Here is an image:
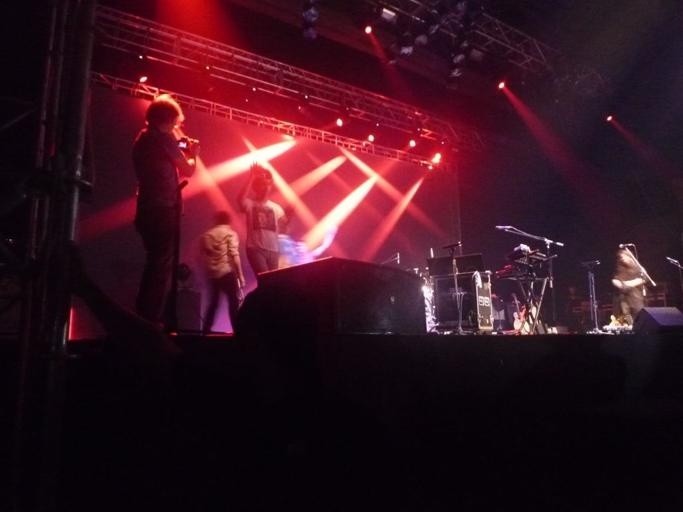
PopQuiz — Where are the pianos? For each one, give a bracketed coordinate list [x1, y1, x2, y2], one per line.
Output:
[498, 248, 546, 279]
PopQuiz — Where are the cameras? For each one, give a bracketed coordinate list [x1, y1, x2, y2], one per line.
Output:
[177, 137, 190, 150]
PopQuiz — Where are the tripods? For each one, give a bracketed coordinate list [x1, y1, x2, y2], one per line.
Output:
[587, 273, 613, 334]
[441, 249, 474, 335]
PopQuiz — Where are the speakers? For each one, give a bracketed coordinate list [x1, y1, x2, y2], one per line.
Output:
[257, 257, 426, 334]
[631, 306, 683, 334]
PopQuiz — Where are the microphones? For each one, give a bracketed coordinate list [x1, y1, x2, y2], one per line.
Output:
[665, 256, 680, 264]
[619, 243, 633, 248]
[496, 226, 512, 230]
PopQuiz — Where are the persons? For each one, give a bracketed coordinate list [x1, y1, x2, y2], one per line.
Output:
[611, 248, 650, 332]
[129, 95, 200, 335]
[202, 210, 246, 333]
[236, 161, 287, 277]
